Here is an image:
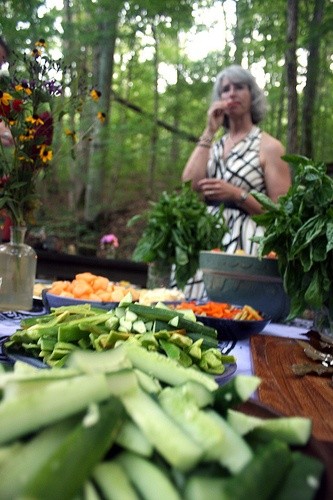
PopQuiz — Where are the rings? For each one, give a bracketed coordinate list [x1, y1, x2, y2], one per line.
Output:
[3, 131, 8, 138]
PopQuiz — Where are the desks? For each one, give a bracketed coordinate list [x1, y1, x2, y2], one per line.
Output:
[0, 312, 333, 500]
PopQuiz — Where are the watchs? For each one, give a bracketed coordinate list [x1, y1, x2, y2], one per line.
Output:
[235, 190, 248, 207]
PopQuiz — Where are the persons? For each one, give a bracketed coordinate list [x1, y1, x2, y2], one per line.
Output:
[170, 65, 291, 307]
[0, 37, 15, 152]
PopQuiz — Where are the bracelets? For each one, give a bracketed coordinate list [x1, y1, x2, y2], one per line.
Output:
[197, 144, 210, 148]
[199, 136, 212, 142]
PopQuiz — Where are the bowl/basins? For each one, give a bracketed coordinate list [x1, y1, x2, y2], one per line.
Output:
[199, 251, 296, 322]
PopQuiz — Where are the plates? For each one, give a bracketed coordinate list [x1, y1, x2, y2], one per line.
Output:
[180, 304, 272, 337]
[2, 339, 238, 380]
[234, 393, 333, 500]
[151, 298, 190, 306]
[45, 292, 140, 314]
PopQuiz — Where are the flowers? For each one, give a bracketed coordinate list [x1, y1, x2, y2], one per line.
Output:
[0, 36, 108, 288]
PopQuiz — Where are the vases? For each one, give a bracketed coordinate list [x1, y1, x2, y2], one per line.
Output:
[0, 224, 39, 315]
[313, 300, 333, 344]
